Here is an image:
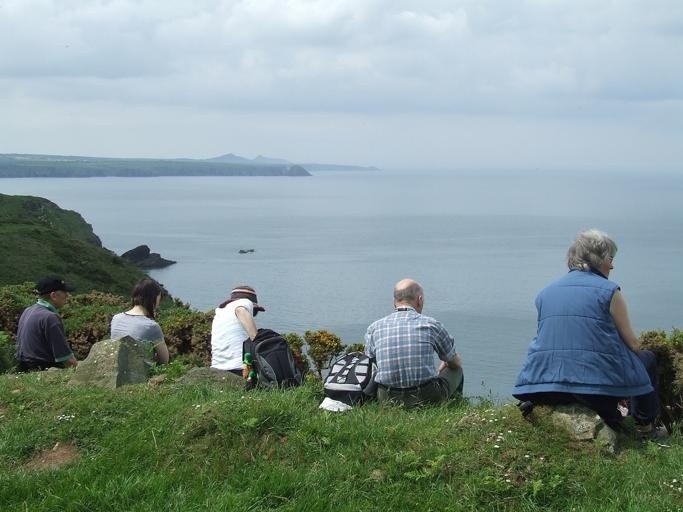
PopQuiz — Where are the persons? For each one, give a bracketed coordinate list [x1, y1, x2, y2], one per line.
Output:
[363, 277, 464, 409]
[14, 274, 78, 373]
[209, 285, 266, 377]
[509, 227, 669, 447]
[111, 277, 170, 366]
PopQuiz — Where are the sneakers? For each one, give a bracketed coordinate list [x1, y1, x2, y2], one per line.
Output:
[635, 423, 667, 443]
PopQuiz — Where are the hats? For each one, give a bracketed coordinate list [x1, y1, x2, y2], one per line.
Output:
[219, 286, 265, 312]
[36, 276, 75, 294]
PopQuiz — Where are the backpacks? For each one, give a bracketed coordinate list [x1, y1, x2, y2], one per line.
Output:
[242, 328, 303, 392]
[323, 350, 377, 407]
